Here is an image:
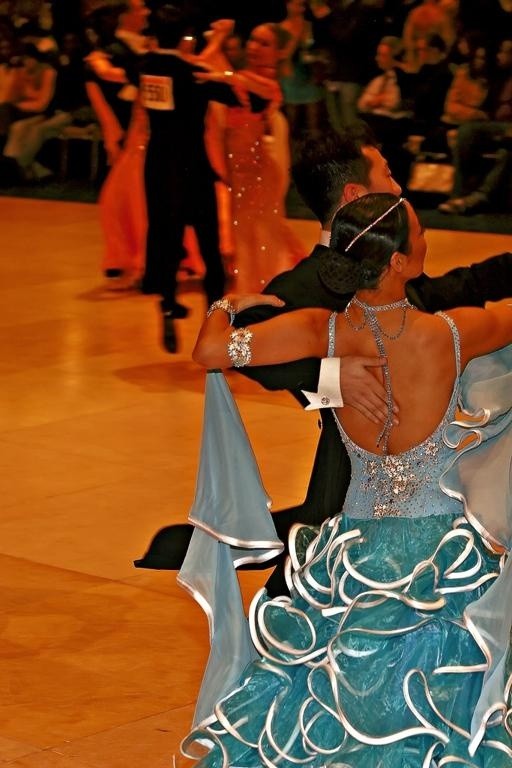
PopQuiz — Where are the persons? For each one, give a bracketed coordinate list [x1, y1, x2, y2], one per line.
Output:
[81, 0, 321, 354]
[191, 190, 512, 768]
[0, 0, 511, 222]
[130, 132, 511, 593]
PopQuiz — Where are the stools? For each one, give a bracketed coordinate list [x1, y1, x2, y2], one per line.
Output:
[59, 125, 103, 195]
